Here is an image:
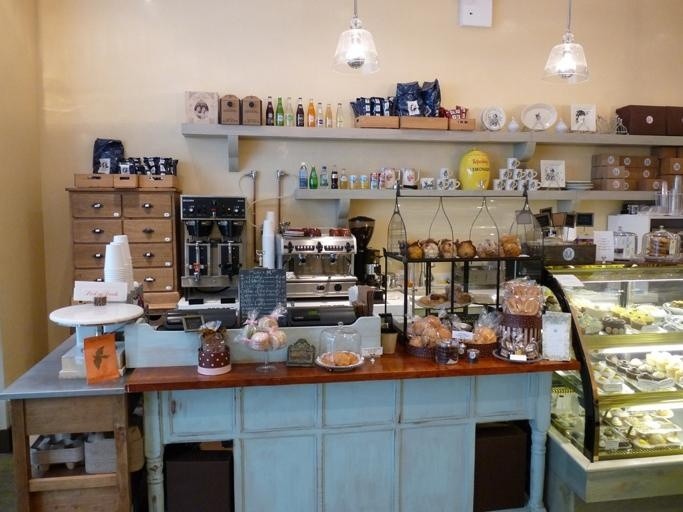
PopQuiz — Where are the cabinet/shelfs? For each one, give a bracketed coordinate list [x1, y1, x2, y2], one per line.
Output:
[66, 188, 183, 327]
[125, 354, 578, 512]
[0, 331, 142, 512]
[542, 260, 683, 512]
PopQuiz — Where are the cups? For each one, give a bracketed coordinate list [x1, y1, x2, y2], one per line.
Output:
[426, 177, 434, 190]
[493, 178, 505, 191]
[437, 179, 449, 192]
[440, 167, 454, 180]
[499, 168, 512, 181]
[524, 169, 538, 180]
[517, 180, 527, 192]
[448, 178, 461, 191]
[512, 168, 526, 180]
[381, 332, 399, 355]
[262, 219, 275, 269]
[507, 158, 520, 169]
[527, 179, 542, 192]
[384, 167, 400, 189]
[504, 179, 517, 192]
[419, 177, 428, 189]
[104, 234, 135, 295]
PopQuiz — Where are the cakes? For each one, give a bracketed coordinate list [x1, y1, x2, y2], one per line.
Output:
[557, 409, 681, 449]
[576, 300, 682, 333]
[596, 356, 682, 392]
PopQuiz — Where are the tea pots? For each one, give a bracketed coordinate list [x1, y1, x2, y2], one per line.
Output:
[401, 167, 420, 189]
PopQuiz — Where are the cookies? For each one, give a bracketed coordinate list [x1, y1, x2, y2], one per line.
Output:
[315, 350, 359, 368]
[244, 316, 285, 350]
[472, 326, 498, 345]
[648, 237, 669, 256]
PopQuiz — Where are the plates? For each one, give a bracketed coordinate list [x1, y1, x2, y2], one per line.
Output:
[599, 327, 630, 335]
[315, 350, 365, 372]
[597, 382, 635, 396]
[642, 254, 683, 265]
[520, 102, 558, 131]
[598, 360, 677, 393]
[642, 358, 683, 390]
[246, 344, 288, 374]
[665, 315, 683, 332]
[663, 300, 683, 315]
[613, 256, 645, 265]
[482, 104, 506, 131]
[637, 303, 671, 319]
[626, 323, 668, 334]
[566, 180, 595, 190]
[492, 348, 542, 364]
[30, 436, 85, 464]
[416, 293, 475, 309]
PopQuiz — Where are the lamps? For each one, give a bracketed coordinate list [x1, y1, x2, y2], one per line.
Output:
[546, 0, 589, 84]
[334, 0, 380, 72]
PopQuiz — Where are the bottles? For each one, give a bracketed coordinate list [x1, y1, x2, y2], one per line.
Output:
[467, 350, 478, 362]
[326, 104, 332, 127]
[287, 97, 292, 126]
[557, 119, 567, 131]
[318, 102, 323, 126]
[331, 164, 338, 188]
[266, 96, 274, 125]
[311, 165, 316, 188]
[437, 342, 449, 363]
[351, 174, 357, 189]
[361, 175, 368, 188]
[341, 168, 347, 189]
[320, 165, 328, 188]
[337, 103, 343, 128]
[296, 97, 304, 126]
[509, 116, 518, 130]
[308, 99, 315, 127]
[299, 161, 308, 188]
[276, 97, 283, 126]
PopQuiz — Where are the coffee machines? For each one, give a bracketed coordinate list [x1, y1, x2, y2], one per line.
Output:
[176, 194, 248, 329]
[348, 216, 384, 304]
[276, 231, 358, 298]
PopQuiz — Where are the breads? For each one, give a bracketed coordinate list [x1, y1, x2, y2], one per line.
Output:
[422, 240, 439, 259]
[502, 236, 521, 257]
[506, 285, 541, 316]
[407, 241, 422, 260]
[458, 240, 475, 258]
[441, 240, 455, 257]
[419, 293, 470, 305]
[406, 314, 452, 347]
